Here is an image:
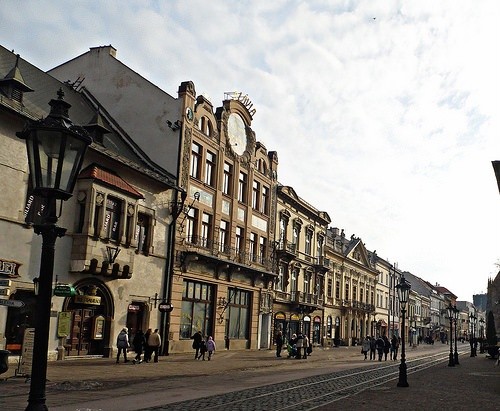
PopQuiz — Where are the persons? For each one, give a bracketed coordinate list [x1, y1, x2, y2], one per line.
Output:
[115, 327, 130, 365]
[307, 342, 313, 355]
[131, 329, 146, 365]
[293, 332, 305, 358]
[288, 333, 297, 347]
[361, 325, 465, 360]
[197, 343, 207, 360]
[303, 334, 309, 358]
[206, 336, 217, 361]
[146, 328, 161, 362]
[275, 331, 283, 357]
[142, 328, 154, 362]
[190, 331, 206, 358]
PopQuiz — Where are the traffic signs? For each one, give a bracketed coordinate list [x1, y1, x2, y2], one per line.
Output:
[0, 298, 25, 307]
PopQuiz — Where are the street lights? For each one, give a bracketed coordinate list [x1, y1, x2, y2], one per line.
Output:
[393, 277, 413, 388]
[451, 306, 460, 365]
[13, 86, 93, 411]
[445, 302, 456, 367]
[469, 312, 486, 356]
